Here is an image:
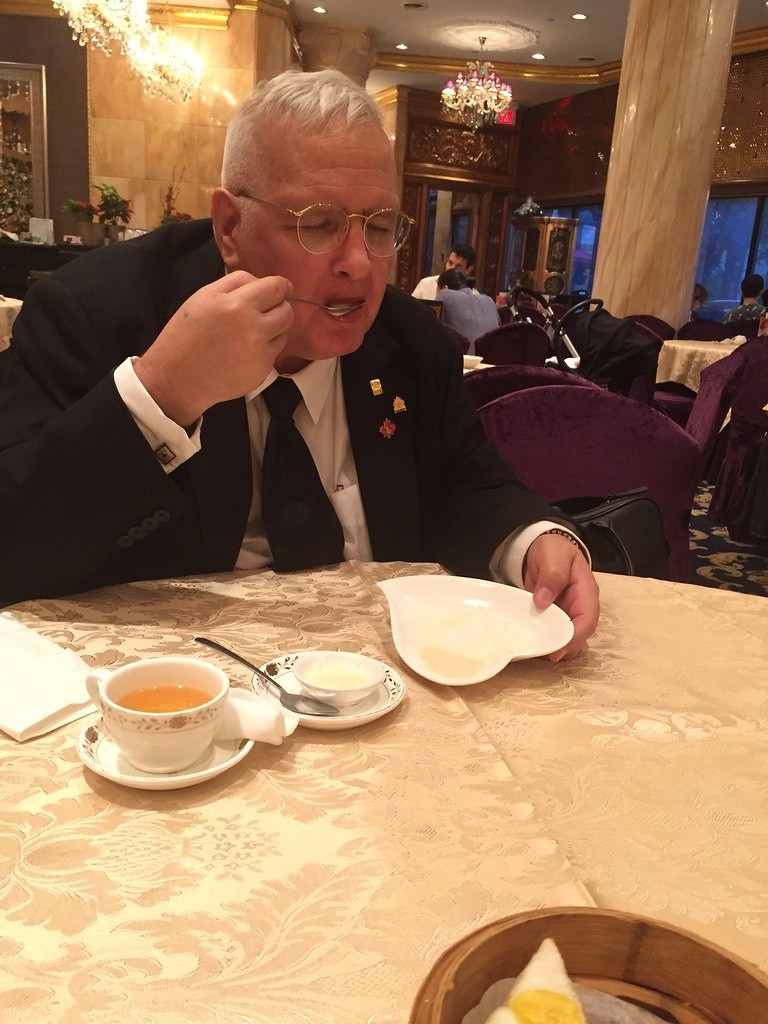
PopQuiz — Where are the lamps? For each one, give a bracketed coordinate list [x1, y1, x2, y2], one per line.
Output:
[441, 32, 512, 129]
[51, 0, 205, 106]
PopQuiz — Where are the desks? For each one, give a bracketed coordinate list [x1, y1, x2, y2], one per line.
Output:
[0, 560, 768, 1022]
[656, 340, 745, 433]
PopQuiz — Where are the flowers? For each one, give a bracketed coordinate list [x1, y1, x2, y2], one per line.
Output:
[62, 184, 134, 228]
[152, 163, 195, 231]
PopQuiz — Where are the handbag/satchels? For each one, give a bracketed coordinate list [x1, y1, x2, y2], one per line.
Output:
[551, 486, 669, 576]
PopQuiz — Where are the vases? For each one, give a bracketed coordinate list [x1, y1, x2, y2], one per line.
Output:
[78, 222, 108, 246]
[108, 223, 125, 245]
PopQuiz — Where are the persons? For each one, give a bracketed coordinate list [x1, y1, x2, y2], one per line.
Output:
[435, 268, 501, 356]
[410, 244, 475, 301]
[689, 273, 768, 324]
[0, 70, 599, 665]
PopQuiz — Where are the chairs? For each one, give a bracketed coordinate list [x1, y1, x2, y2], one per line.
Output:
[415, 299, 768, 584]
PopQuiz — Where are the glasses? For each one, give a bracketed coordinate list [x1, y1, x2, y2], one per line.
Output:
[233, 192, 417, 258]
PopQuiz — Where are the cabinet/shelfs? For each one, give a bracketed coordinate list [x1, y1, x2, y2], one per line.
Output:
[0, 61, 50, 236]
[505, 214, 579, 314]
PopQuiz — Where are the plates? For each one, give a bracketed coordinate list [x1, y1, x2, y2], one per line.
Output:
[252, 651, 406, 730]
[377, 575, 575, 686]
[76, 716, 255, 790]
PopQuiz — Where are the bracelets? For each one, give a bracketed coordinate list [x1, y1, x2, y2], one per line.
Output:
[543, 529, 579, 548]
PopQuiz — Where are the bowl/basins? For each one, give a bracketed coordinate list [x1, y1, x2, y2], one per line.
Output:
[463, 355, 483, 368]
[293, 654, 385, 704]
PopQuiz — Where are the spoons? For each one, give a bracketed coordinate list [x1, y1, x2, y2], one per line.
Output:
[195, 637, 340, 715]
[287, 297, 367, 316]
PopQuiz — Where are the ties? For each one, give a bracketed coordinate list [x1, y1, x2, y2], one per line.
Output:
[261, 376, 345, 573]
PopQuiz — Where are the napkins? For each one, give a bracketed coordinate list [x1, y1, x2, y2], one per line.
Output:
[0, 610, 100, 742]
[211, 686, 300, 746]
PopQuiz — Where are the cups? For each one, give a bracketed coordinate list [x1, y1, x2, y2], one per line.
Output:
[85, 657, 229, 774]
